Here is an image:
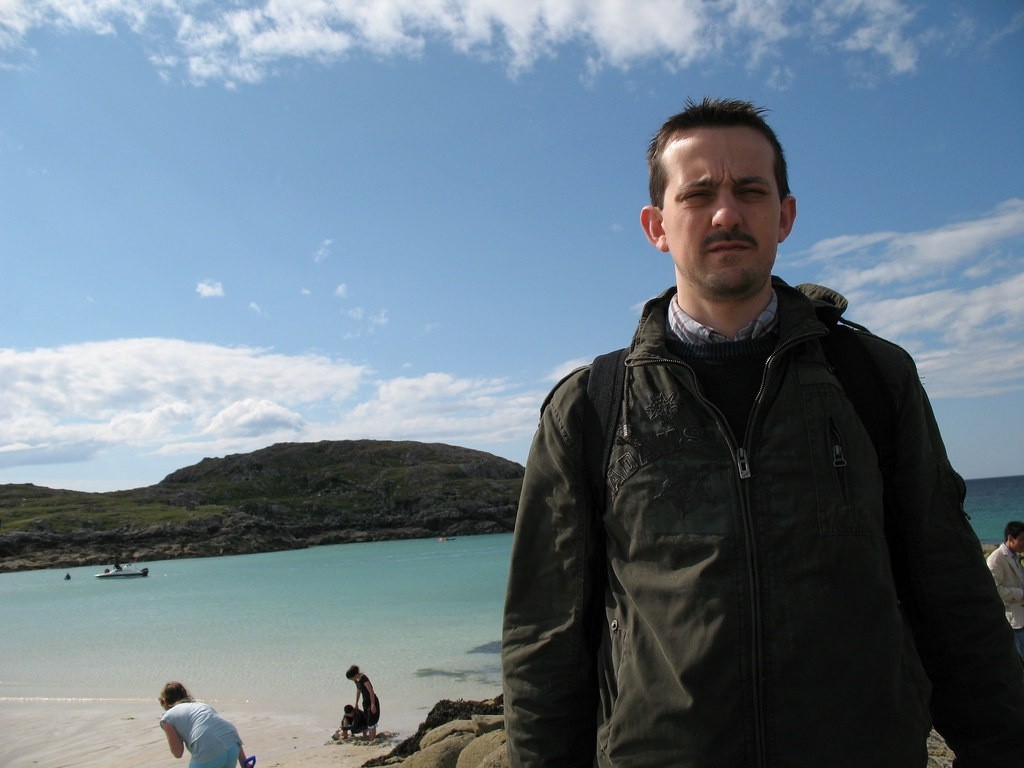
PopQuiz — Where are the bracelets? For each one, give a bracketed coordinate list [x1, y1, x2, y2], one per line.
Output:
[371, 705, 375, 707]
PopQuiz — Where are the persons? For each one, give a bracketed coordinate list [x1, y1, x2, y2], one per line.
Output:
[340, 665, 380, 742]
[501, 100, 1024, 767]
[159, 681, 253, 768]
[984, 520, 1023, 660]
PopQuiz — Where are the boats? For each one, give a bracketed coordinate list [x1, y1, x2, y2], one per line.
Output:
[94, 565, 149, 578]
